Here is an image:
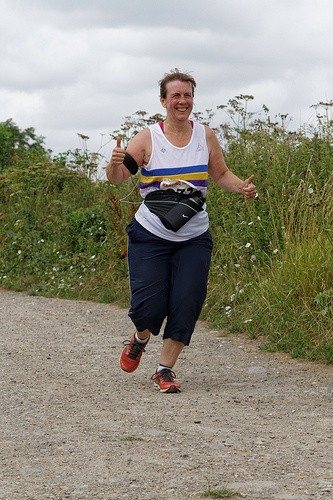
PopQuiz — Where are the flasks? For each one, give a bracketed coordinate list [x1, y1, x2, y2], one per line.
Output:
[163, 189, 206, 233]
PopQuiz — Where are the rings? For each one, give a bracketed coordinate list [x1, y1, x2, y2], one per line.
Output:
[250, 194, 252, 198]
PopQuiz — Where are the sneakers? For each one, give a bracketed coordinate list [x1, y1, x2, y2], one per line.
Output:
[119, 330, 150, 373]
[152, 368, 182, 394]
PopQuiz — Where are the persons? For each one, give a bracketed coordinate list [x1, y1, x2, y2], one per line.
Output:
[105, 72, 258, 392]
